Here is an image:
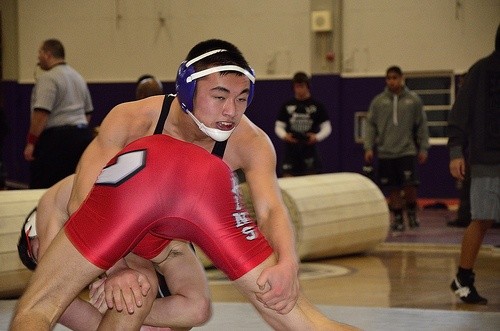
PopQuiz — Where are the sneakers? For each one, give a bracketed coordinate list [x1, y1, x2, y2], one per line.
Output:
[450, 275, 488, 305]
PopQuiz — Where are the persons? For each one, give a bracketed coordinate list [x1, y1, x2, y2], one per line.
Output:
[449, 24, 500, 305]
[67, 39, 300, 331]
[447, 73, 472, 228]
[362, 66, 429, 231]
[8, 135, 362, 331]
[24, 38, 93, 189]
[133, 75, 162, 100]
[274, 71, 332, 178]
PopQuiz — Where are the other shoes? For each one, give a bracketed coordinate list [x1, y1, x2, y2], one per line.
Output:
[447, 216, 471, 227]
[408, 210, 420, 228]
[390, 214, 404, 236]
